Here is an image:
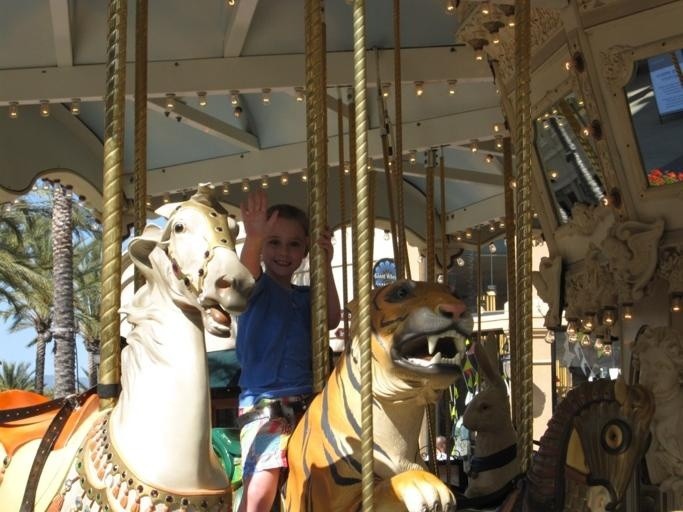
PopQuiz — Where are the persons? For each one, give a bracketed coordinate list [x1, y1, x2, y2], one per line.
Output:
[233, 189, 341, 512]
[424, 436, 454, 461]
